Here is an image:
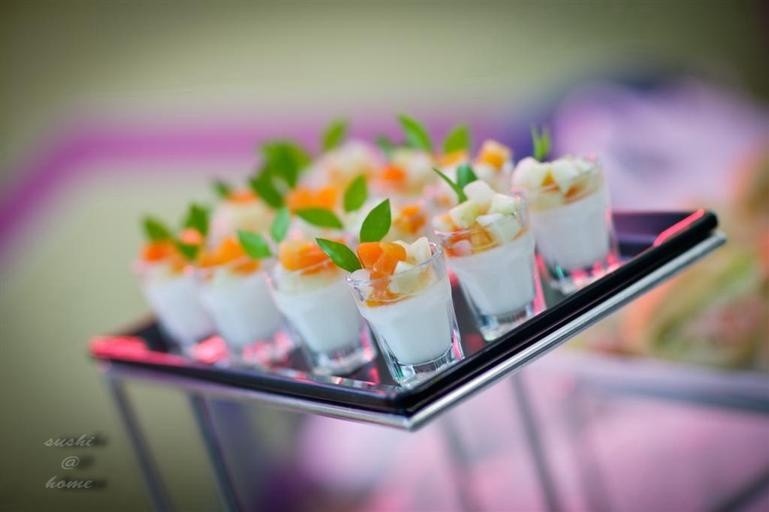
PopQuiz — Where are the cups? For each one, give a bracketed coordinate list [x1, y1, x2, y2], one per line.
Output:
[345, 241, 465, 385]
[511, 164, 621, 296]
[434, 195, 548, 345]
[139, 142, 513, 375]
